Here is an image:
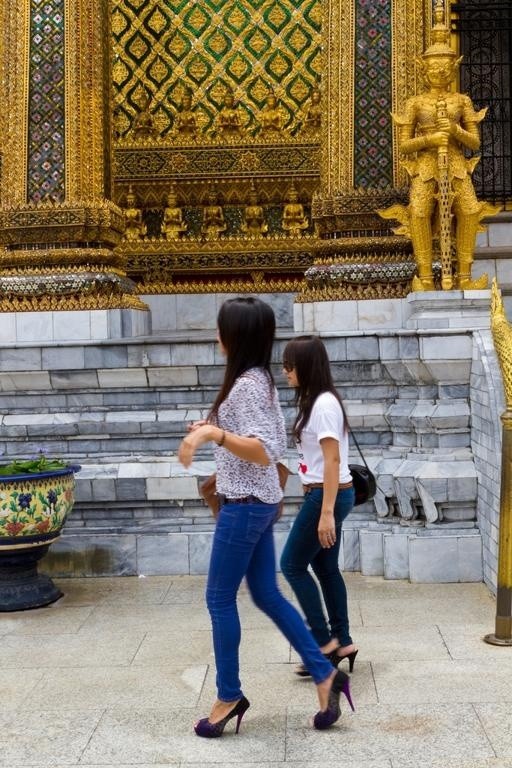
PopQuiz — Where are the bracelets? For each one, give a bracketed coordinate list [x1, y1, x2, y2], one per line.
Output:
[216, 430, 226, 447]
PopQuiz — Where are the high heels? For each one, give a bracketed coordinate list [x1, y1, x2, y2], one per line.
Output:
[314, 670, 354, 729]
[193, 694, 250, 739]
[295, 643, 339, 676]
[332, 648, 358, 673]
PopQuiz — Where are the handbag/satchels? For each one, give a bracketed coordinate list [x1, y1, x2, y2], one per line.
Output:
[201, 461, 289, 525]
[348, 464, 377, 506]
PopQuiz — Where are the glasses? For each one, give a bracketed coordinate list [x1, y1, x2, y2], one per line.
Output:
[283, 360, 296, 373]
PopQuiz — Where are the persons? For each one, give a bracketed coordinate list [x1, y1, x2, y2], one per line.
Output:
[300, 89, 324, 138]
[122, 185, 147, 241]
[280, 187, 309, 234]
[175, 296, 357, 739]
[132, 89, 158, 142]
[217, 92, 244, 143]
[260, 92, 287, 139]
[172, 91, 198, 141]
[160, 184, 187, 237]
[375, 2, 506, 293]
[277, 335, 359, 677]
[200, 189, 230, 238]
[240, 187, 270, 236]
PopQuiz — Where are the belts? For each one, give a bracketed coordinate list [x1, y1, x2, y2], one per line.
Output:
[302, 481, 353, 495]
[224, 498, 264, 504]
